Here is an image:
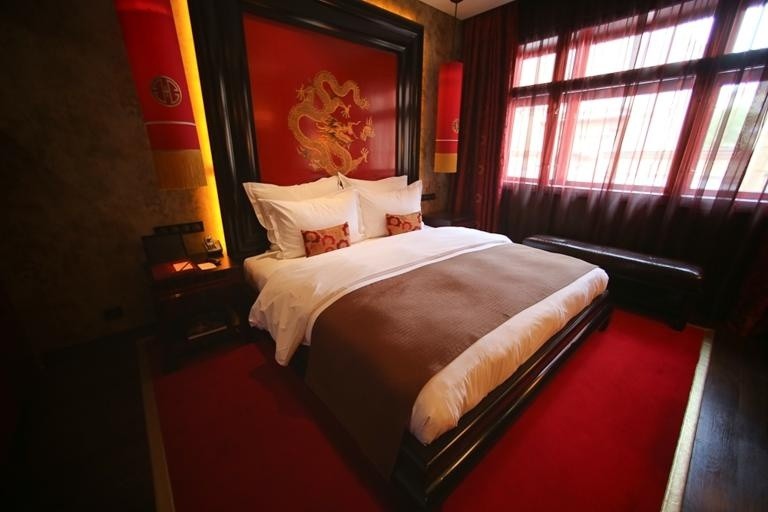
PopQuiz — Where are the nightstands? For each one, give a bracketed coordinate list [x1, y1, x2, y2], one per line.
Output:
[423, 210, 477, 229]
[140, 254, 248, 371]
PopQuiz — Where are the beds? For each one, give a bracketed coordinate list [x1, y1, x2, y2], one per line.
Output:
[188, 0, 614, 512]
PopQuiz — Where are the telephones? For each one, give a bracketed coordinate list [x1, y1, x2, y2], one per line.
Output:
[200, 235, 222, 257]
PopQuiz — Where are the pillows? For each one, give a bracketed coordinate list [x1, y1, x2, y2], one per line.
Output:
[242, 171, 422, 259]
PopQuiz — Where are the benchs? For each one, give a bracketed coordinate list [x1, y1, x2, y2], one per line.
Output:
[522, 234, 706, 331]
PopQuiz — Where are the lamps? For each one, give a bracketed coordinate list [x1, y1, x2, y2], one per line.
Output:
[114, 0, 207, 190]
[433, 0, 464, 173]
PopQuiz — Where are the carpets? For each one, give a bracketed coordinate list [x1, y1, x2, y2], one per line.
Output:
[135, 304, 715, 512]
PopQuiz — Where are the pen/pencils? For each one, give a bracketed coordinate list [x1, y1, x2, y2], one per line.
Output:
[180, 261, 191, 272]
